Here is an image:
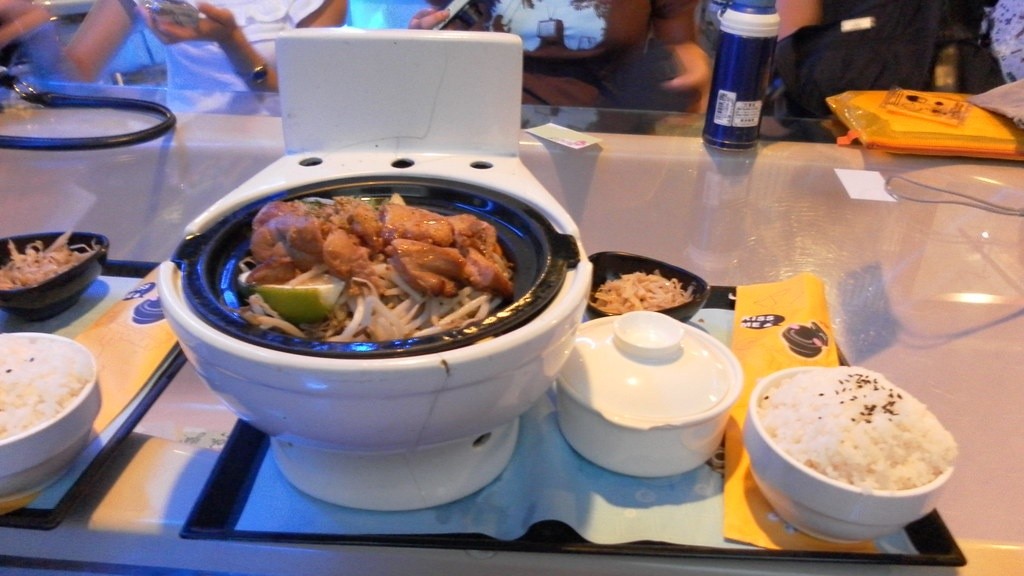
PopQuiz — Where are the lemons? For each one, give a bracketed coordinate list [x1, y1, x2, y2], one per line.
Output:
[253, 282, 345, 322]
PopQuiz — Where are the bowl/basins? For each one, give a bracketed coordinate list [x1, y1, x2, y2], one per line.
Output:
[744, 366, 955, 544]
[173, 177, 580, 362]
[0, 332, 101, 500]
[589, 251, 713, 324]
[556, 310, 744, 478]
[0, 231, 110, 322]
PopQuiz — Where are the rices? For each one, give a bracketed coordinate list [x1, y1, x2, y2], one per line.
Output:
[759, 365, 958, 492]
[0, 331, 94, 442]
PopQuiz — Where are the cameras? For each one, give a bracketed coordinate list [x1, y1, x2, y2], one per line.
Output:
[146, 0, 199, 28]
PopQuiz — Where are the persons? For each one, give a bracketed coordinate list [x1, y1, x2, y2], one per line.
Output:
[407, 2, 1024, 136]
[0, 0, 350, 93]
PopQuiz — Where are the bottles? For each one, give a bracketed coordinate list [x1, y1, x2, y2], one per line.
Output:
[703, 0, 781, 151]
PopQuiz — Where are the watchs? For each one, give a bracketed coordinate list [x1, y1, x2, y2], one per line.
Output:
[233, 62, 268, 85]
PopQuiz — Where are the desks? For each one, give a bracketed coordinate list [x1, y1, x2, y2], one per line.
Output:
[0, 74, 1024, 576]
